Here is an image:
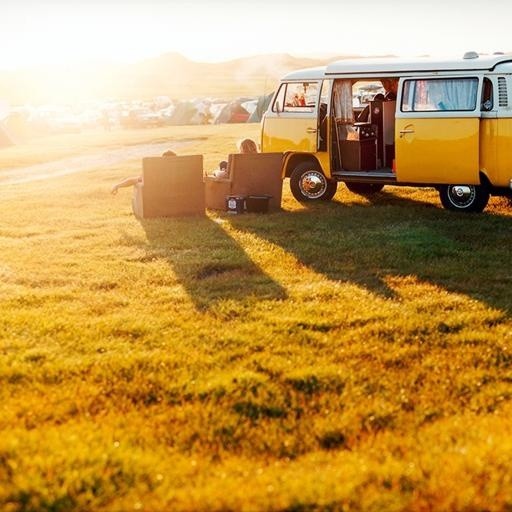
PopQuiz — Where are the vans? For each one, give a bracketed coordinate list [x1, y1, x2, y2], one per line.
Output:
[259, 56, 512, 214]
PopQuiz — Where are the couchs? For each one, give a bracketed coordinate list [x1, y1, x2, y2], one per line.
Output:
[132, 155, 206, 218]
[205, 152, 283, 211]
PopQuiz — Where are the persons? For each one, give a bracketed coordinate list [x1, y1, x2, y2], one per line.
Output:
[239, 138, 258, 154]
[110, 150, 176, 196]
